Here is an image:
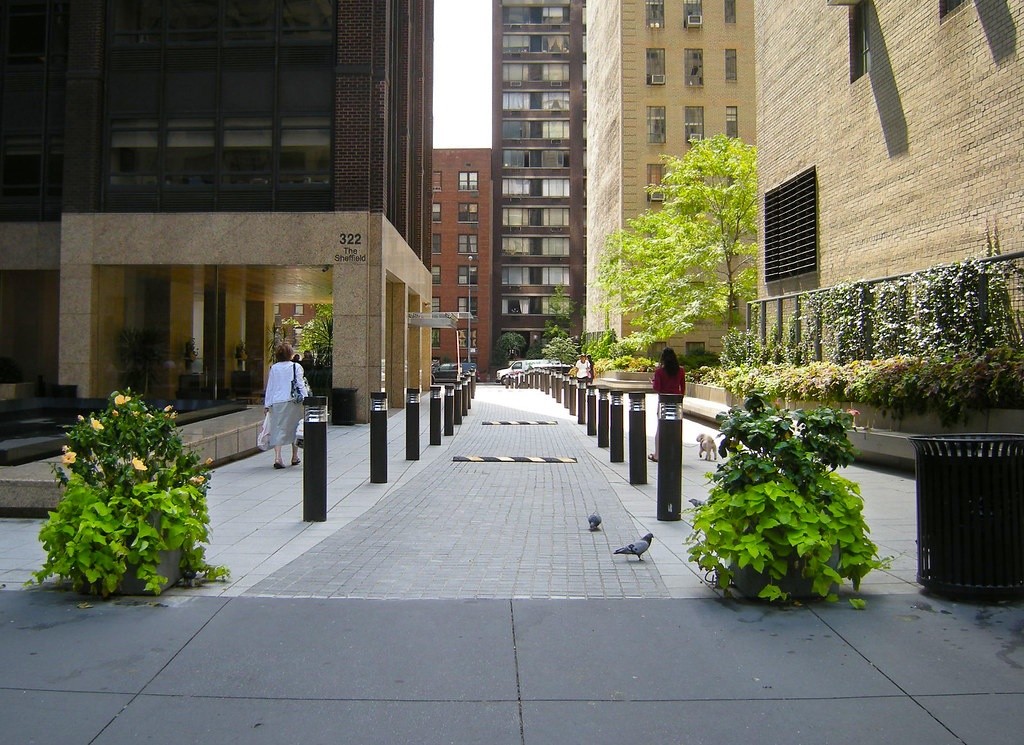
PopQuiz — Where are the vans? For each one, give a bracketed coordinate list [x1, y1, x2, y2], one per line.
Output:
[496, 360, 562, 385]
[431, 363, 477, 385]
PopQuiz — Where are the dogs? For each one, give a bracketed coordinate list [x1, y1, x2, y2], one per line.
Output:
[696, 434, 717, 461]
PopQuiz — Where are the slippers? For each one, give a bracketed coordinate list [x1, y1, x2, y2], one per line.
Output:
[648, 453, 659, 463]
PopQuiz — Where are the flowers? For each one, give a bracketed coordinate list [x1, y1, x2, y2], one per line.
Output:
[22, 387, 233, 598]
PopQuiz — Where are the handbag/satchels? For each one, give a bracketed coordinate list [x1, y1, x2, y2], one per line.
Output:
[257, 411, 271, 451]
[294, 418, 305, 449]
[290, 362, 312, 404]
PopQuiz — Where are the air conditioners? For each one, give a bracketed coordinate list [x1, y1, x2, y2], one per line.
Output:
[686, 14, 703, 30]
[550, 80, 562, 87]
[509, 80, 522, 87]
[648, 188, 667, 202]
[650, 74, 666, 85]
[689, 133, 702, 144]
[551, 138, 563, 146]
[508, 195, 521, 204]
[509, 225, 522, 233]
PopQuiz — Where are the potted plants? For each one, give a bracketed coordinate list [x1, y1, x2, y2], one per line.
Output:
[693, 408, 889, 603]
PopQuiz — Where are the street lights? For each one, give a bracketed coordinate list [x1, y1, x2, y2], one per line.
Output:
[467, 256, 472, 365]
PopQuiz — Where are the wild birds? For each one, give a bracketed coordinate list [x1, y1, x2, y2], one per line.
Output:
[587, 511, 602, 531]
[613, 532, 654, 561]
[689, 498, 708, 512]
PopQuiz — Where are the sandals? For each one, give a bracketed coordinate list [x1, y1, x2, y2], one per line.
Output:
[292, 457, 300, 465]
[273, 459, 286, 469]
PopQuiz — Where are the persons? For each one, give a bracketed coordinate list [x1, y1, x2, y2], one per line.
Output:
[292, 351, 313, 362]
[264, 344, 309, 469]
[646, 347, 685, 462]
[569, 354, 595, 385]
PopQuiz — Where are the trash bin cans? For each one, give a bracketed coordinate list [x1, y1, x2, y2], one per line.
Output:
[907, 432, 1023, 603]
[330, 388, 358, 426]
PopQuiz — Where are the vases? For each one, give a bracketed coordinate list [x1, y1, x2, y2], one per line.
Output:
[70, 528, 197, 597]
